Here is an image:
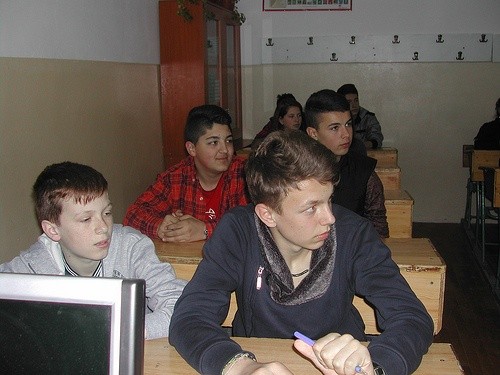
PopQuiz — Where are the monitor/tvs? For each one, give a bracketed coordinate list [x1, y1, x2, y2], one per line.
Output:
[1, 271, 146, 375]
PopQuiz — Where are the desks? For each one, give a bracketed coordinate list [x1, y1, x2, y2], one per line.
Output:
[374, 165, 400, 190]
[383, 190, 414, 238]
[366, 148, 398, 164]
[478, 166, 500, 207]
[144, 336, 465, 375]
[152, 239, 446, 336]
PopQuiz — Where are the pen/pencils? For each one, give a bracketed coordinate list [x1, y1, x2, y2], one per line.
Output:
[293, 331, 370, 375]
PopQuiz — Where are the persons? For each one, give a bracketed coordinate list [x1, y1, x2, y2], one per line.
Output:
[273, 99, 307, 134]
[0, 162, 190, 342]
[122, 105, 253, 243]
[251, 93, 297, 150]
[304, 89, 390, 239]
[473, 98, 500, 219]
[168, 130, 434, 375]
[336, 84, 383, 150]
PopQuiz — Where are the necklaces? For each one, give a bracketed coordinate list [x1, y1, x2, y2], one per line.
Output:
[291, 269, 310, 277]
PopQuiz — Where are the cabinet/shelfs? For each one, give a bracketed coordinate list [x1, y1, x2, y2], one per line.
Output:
[158, 0, 243, 170]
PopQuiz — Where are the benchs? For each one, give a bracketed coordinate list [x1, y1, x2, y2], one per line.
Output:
[471, 151, 499, 262]
[462, 146, 478, 228]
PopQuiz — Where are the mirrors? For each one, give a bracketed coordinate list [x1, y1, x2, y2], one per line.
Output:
[226, 25, 237, 130]
[207, 18, 220, 109]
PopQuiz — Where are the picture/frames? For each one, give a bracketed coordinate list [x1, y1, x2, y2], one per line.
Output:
[263, 0, 352, 11]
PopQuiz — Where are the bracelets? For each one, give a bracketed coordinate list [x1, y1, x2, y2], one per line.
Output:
[220, 352, 257, 374]
[204, 225, 208, 241]
[372, 362, 386, 375]
